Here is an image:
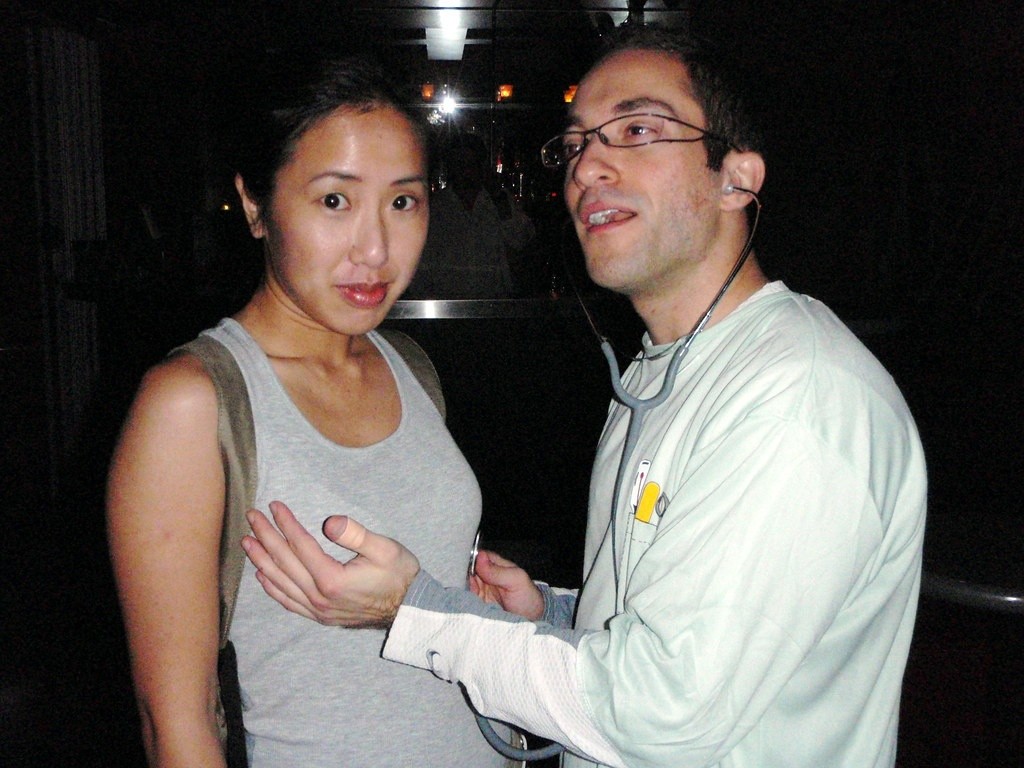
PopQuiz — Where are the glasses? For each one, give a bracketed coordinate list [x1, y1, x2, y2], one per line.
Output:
[540, 113, 743, 168]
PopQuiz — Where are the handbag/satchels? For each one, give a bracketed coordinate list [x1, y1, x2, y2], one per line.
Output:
[215, 641, 248, 768]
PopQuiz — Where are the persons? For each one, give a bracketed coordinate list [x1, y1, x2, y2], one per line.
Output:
[238, 26, 927, 768]
[103, 58, 518, 767]
